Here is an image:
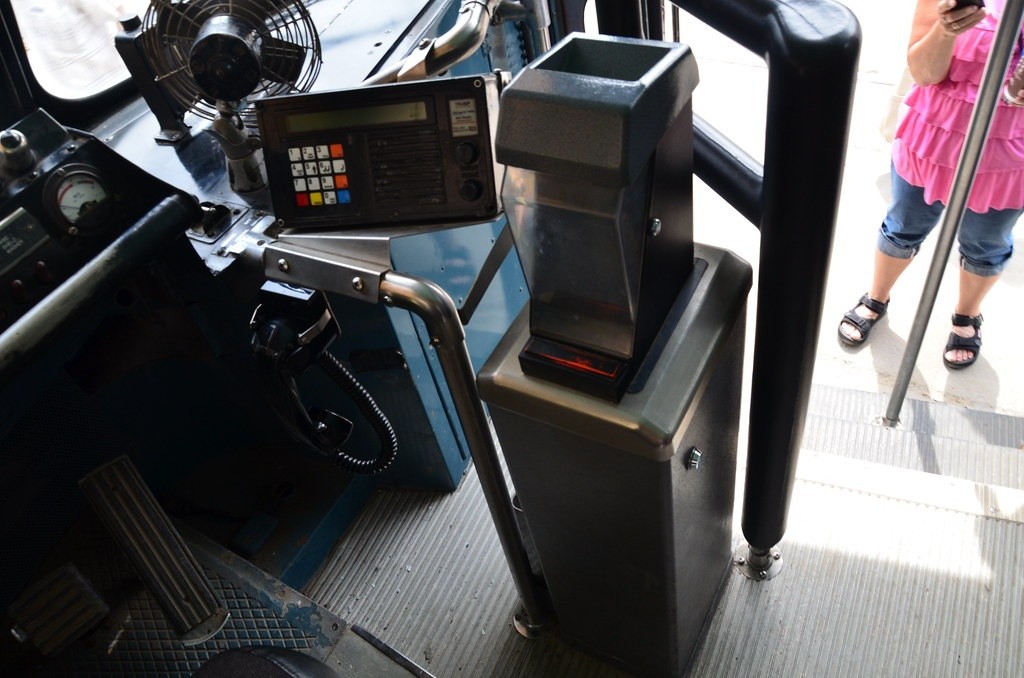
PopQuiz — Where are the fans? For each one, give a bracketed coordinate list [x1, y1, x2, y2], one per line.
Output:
[141, 0, 324, 193]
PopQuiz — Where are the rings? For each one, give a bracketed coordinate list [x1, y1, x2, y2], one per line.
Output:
[951, 21, 960, 30]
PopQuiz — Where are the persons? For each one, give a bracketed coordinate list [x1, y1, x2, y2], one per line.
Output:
[837, 0, 1024, 371]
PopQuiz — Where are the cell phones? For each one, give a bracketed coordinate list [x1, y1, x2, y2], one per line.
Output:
[953, 0, 986, 10]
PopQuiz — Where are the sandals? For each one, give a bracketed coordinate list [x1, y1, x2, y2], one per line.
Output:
[943, 308, 984, 369]
[838, 292, 890, 346]
